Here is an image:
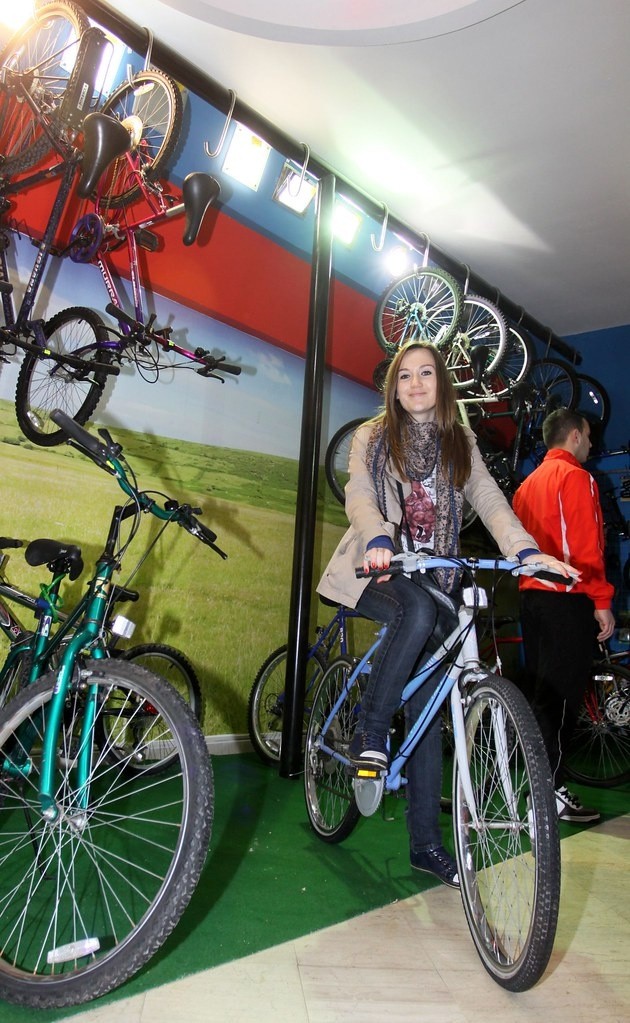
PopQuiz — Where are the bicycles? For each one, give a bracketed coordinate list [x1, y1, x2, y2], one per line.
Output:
[0, 536, 207, 776]
[301, 548, 576, 993]
[0, 407, 229, 1007]
[248, 593, 514, 816]
[475, 600, 630, 789]
[326, 266, 612, 535]
[0, 0, 242, 446]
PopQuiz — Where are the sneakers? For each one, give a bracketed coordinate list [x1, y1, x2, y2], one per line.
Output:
[554, 788, 601, 820]
[411, 841, 464, 887]
[344, 726, 388, 771]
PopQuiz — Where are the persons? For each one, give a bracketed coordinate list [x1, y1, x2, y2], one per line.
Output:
[510, 409, 614, 823]
[314, 342, 583, 890]
